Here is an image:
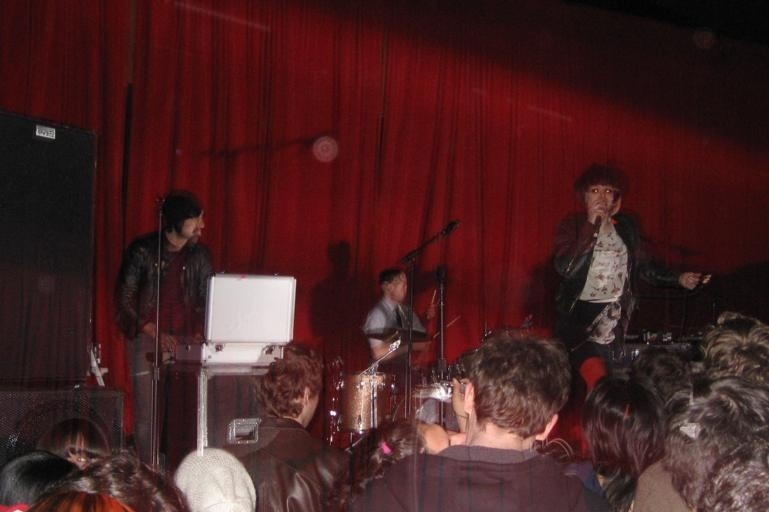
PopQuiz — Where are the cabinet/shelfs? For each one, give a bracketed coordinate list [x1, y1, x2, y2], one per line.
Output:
[171, 365, 273, 457]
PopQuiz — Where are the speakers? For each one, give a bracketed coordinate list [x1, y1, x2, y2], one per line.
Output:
[0, 112, 94, 267]
[0, 386, 123, 469]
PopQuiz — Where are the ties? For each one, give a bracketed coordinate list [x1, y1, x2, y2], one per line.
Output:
[395, 306, 402, 329]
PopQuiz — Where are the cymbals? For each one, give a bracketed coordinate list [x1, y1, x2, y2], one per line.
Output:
[363, 326, 433, 343]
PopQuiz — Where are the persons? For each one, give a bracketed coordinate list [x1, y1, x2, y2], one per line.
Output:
[345, 330, 611, 511]
[113, 193, 214, 466]
[355, 268, 429, 387]
[168, 449, 257, 511]
[579, 313, 768, 512]
[0, 420, 189, 512]
[220, 340, 367, 511]
[320, 420, 465, 511]
[546, 163, 710, 382]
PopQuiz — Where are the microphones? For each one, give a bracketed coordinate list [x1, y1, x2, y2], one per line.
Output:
[439, 216, 462, 238]
[592, 215, 602, 239]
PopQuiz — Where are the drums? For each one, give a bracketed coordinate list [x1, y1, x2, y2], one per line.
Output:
[461, 346, 485, 375]
[338, 372, 398, 438]
[394, 381, 460, 434]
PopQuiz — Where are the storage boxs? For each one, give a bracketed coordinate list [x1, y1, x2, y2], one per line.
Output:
[174, 275, 297, 363]
